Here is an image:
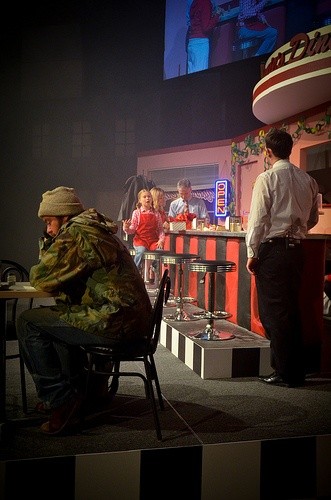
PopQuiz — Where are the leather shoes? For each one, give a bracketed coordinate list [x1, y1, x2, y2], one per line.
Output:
[256, 371, 305, 387]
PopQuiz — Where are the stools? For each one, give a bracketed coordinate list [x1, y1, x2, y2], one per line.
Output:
[141, 249, 176, 310]
[160, 251, 203, 322]
[187, 259, 238, 342]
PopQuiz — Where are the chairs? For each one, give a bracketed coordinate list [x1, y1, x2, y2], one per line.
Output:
[77, 267, 172, 443]
[0, 258, 37, 418]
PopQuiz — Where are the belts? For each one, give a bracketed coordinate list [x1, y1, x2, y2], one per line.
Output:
[267, 240, 301, 244]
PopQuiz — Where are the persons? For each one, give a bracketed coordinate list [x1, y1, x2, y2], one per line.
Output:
[233, 0, 279, 58]
[164, 179, 211, 225]
[14, 186, 155, 437]
[121, 188, 165, 291]
[186, 0, 225, 74]
[148, 187, 165, 285]
[244, 128, 320, 387]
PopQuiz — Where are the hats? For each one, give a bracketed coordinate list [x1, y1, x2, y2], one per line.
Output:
[38, 186, 83, 218]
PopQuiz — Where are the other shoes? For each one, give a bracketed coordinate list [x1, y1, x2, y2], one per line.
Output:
[35, 394, 79, 435]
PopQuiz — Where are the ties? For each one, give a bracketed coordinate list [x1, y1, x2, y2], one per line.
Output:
[184, 200, 189, 217]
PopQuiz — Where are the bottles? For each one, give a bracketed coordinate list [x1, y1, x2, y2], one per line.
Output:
[7, 272, 16, 286]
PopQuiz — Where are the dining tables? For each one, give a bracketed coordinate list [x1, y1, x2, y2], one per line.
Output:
[0, 280, 60, 418]
